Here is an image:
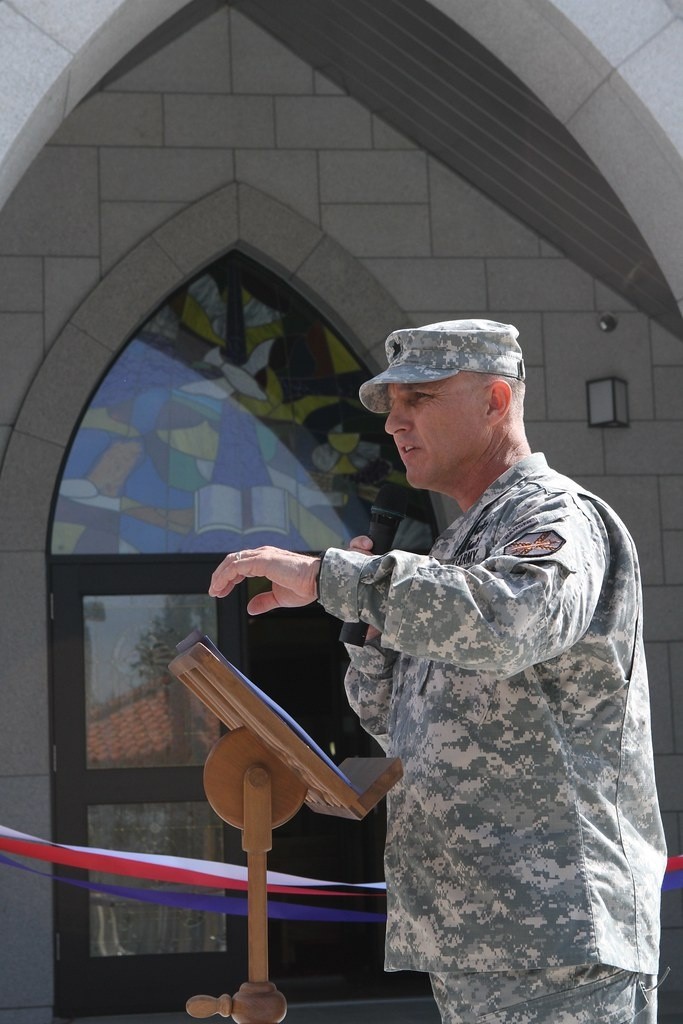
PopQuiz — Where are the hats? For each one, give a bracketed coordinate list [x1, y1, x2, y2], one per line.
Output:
[355, 315, 527, 414]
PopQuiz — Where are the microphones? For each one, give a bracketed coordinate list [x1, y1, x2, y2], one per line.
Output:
[339, 482, 410, 649]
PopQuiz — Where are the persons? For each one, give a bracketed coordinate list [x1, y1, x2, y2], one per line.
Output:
[209, 320, 668, 1024]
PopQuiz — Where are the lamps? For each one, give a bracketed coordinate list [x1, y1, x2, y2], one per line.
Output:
[586, 375, 631, 429]
